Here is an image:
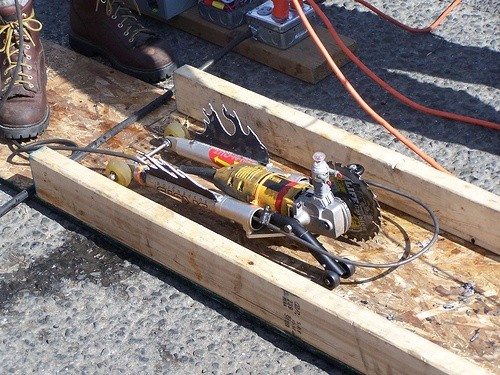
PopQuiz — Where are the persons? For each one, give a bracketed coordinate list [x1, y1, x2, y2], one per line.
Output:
[0, 0, 178, 139]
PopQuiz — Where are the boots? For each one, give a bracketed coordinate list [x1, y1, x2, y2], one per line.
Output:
[68, 0, 178, 80]
[0, 0, 51, 139]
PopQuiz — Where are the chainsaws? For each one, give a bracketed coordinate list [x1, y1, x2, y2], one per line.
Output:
[213, 152, 382, 242]
